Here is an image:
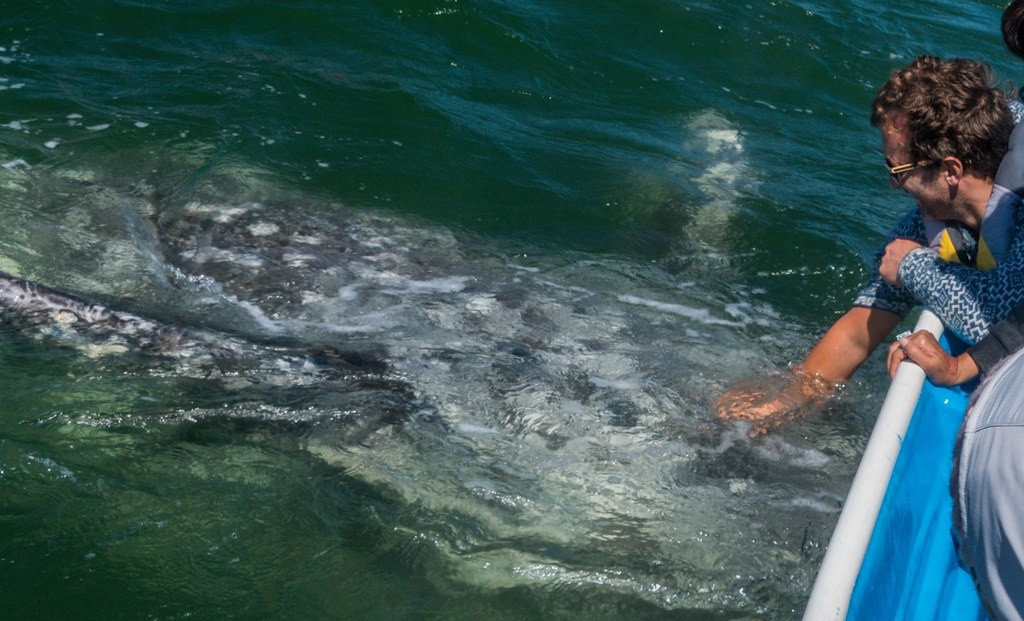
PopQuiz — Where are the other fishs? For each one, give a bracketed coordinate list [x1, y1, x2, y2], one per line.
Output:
[0, 106, 891, 621]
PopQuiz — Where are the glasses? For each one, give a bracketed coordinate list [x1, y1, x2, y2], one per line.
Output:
[884, 159, 935, 188]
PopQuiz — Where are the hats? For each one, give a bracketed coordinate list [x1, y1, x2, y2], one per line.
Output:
[1001, 0, 1024, 58]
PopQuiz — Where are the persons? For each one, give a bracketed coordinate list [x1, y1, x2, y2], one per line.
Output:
[796, 0, 1023, 621]
